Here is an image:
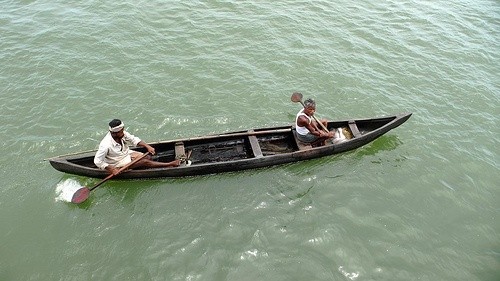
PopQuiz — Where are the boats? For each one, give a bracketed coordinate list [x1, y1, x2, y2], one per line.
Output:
[48, 111, 414, 204]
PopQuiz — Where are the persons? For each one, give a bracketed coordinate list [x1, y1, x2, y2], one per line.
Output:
[93, 119, 183, 176]
[295, 97, 336, 148]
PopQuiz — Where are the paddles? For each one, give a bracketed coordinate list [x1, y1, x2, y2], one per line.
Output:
[71, 151, 150, 203]
[292, 92, 330, 133]
